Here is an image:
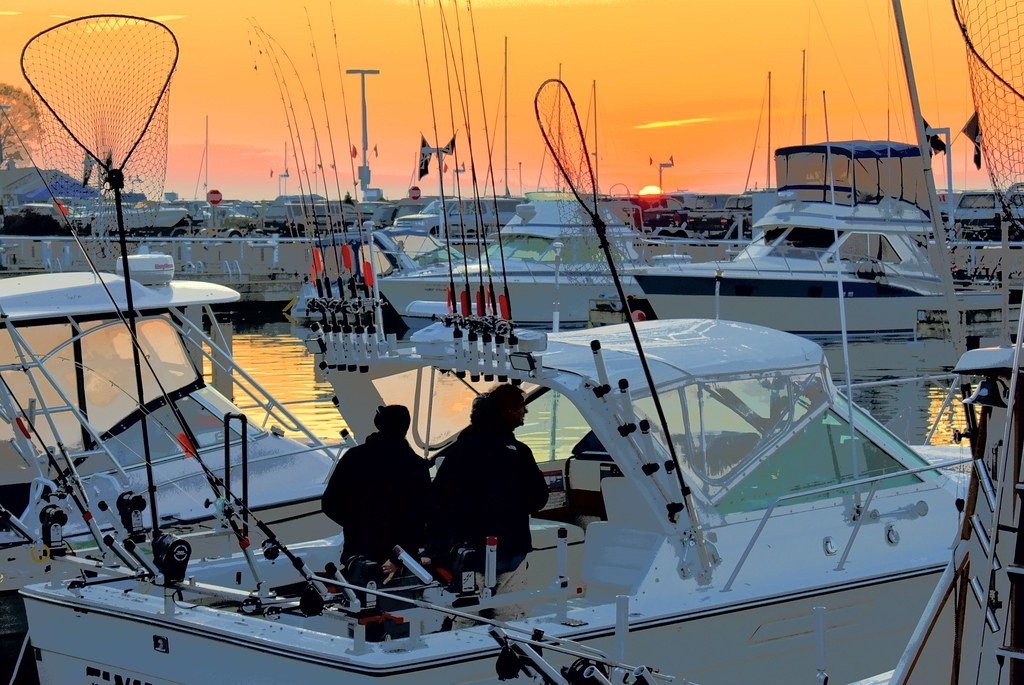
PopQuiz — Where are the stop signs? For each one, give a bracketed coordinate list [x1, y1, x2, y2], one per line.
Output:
[207, 190, 223, 206]
[410, 186, 422, 202]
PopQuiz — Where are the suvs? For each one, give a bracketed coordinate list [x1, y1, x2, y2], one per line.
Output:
[394, 198, 494, 240]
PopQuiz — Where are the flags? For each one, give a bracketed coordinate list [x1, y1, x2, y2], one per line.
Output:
[962, 111, 983, 170]
[441, 133, 457, 156]
[418, 133, 432, 181]
[461, 162, 465, 171]
[922, 116, 947, 156]
[350, 144, 358, 159]
[650, 157, 653, 166]
[669, 155, 674, 167]
[286, 168, 289, 176]
[374, 144, 378, 158]
[271, 169, 273, 178]
[444, 163, 449, 173]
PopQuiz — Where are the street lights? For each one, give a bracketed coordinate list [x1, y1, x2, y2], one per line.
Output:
[346, 67, 379, 199]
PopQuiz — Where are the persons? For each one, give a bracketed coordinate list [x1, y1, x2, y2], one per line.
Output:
[430, 383, 549, 576]
[320, 406, 432, 587]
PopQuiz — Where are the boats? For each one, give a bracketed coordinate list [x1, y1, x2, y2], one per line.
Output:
[0, 1, 1024, 685]
